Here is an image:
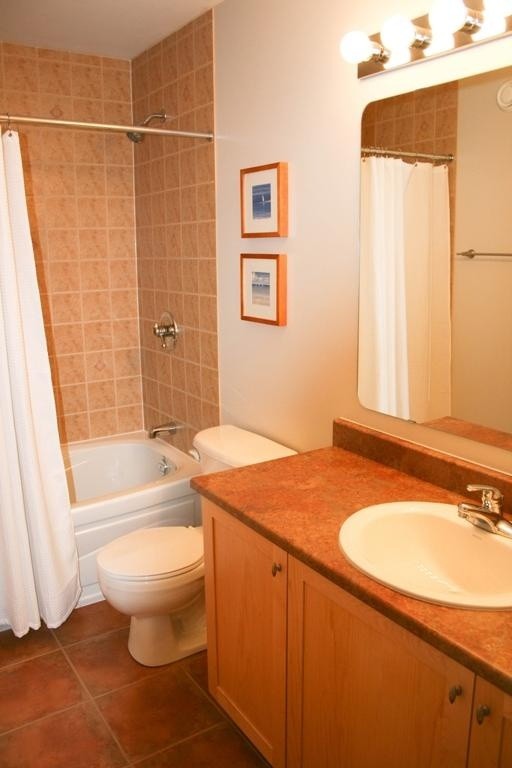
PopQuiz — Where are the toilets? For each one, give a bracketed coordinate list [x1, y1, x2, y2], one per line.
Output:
[95, 426, 302, 670]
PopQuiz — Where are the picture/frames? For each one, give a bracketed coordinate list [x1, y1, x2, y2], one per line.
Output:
[240, 161, 288, 327]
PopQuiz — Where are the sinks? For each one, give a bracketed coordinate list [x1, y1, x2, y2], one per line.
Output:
[337, 501, 512, 613]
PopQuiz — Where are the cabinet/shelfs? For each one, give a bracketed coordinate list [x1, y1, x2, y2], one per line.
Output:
[188, 495, 288, 767]
[289, 555, 512, 768]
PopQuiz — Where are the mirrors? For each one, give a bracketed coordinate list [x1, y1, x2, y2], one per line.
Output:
[355, 65, 512, 453]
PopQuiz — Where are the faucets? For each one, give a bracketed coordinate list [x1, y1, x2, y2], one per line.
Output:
[149, 421, 178, 439]
[456, 483, 512, 539]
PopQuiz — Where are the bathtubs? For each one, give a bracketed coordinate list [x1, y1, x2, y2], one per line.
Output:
[0, 429, 205, 633]
